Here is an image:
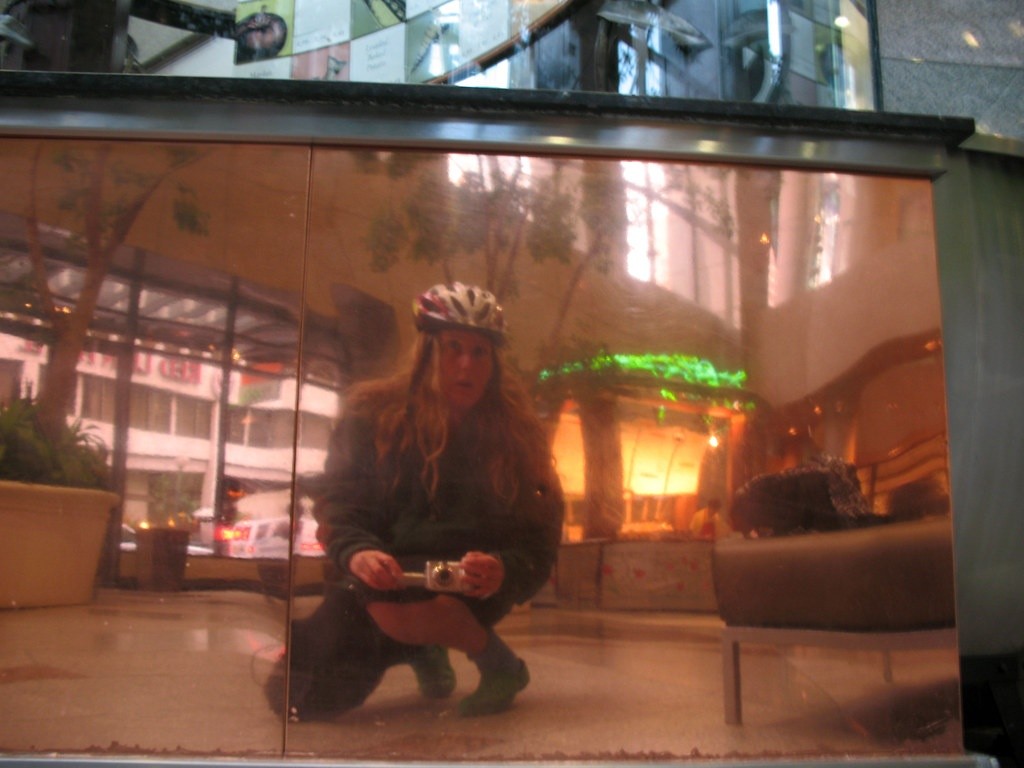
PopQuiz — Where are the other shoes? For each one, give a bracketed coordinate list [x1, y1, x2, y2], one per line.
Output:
[410, 644, 456, 695]
[469, 629, 529, 714]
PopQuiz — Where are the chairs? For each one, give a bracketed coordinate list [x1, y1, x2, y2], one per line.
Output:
[725, 353, 959, 729]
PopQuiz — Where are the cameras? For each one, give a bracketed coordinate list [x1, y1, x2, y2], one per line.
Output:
[400, 560, 474, 593]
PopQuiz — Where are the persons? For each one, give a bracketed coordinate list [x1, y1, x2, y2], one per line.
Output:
[265, 282, 566, 720]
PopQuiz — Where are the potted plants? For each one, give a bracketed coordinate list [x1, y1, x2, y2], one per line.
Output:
[0, 376, 125, 611]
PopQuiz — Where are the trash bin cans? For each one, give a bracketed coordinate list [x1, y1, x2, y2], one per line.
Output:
[133, 526, 189, 592]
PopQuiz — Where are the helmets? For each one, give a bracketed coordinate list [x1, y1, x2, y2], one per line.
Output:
[412, 281, 507, 346]
